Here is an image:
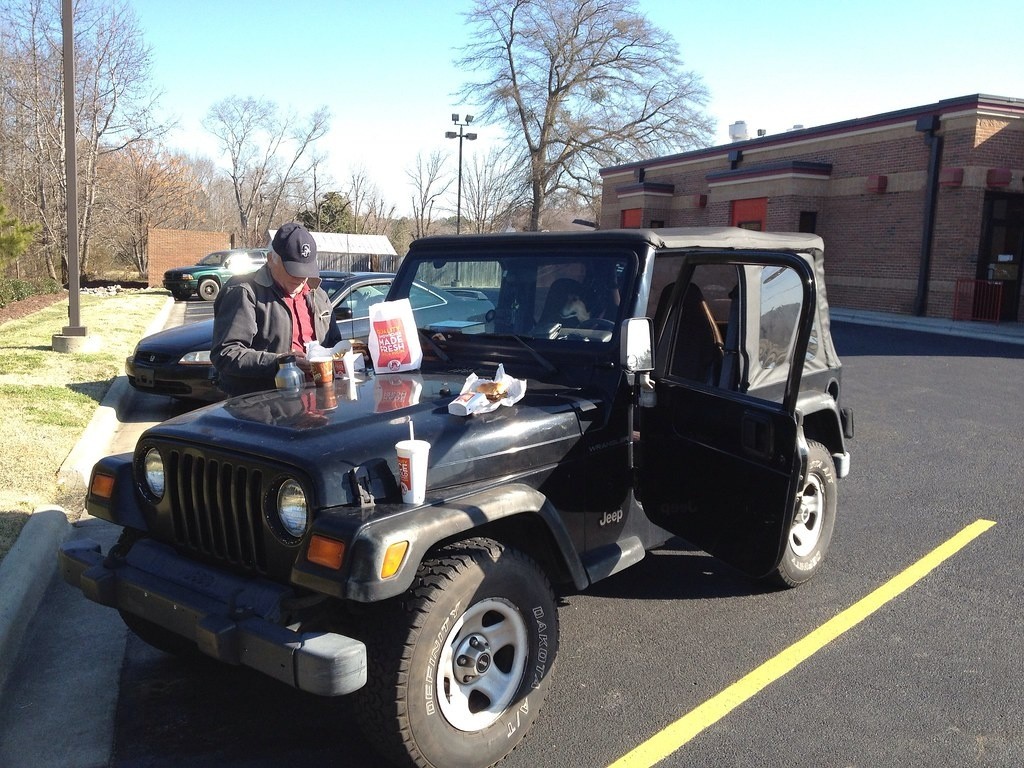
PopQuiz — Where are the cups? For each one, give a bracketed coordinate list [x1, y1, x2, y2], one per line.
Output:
[394, 439, 431, 504]
[316, 387, 338, 411]
[309, 357, 335, 386]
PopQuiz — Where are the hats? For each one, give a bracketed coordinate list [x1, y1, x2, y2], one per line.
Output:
[272, 223, 321, 278]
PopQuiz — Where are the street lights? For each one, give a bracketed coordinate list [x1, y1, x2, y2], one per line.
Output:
[445, 114, 477, 288]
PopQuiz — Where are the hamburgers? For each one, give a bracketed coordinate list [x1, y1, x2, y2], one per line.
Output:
[352, 343, 369, 355]
[477, 382, 509, 403]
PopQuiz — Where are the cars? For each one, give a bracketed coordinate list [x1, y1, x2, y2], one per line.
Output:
[125, 248, 496, 402]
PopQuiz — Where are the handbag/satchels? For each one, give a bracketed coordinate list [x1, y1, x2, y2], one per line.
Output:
[368, 298, 423, 375]
[375, 371, 423, 414]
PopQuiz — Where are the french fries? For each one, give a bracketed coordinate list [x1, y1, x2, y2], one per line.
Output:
[332, 352, 345, 358]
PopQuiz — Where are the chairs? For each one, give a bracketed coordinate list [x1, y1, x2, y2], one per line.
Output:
[540, 278, 587, 328]
[654, 281, 725, 384]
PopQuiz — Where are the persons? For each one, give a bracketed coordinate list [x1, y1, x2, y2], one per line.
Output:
[589, 256, 626, 323]
[209, 223, 342, 396]
[222, 385, 328, 430]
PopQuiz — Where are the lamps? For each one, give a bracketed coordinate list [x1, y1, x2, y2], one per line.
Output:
[757, 129, 766, 137]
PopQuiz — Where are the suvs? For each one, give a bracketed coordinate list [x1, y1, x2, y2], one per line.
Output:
[57, 227, 856, 768]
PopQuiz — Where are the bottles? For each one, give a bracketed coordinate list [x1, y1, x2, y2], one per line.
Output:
[274, 355, 305, 388]
